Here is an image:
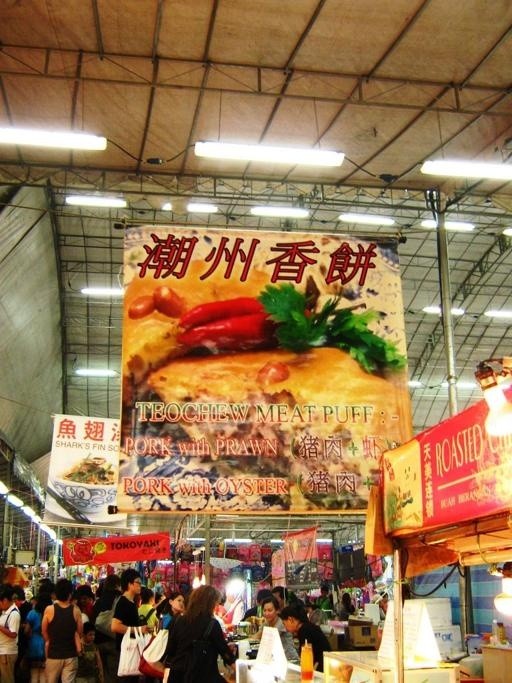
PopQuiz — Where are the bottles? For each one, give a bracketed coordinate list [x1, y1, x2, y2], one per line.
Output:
[300, 638, 314, 682]
[491, 620, 504, 643]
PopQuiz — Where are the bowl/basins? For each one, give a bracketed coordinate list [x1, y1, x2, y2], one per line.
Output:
[51, 475, 119, 513]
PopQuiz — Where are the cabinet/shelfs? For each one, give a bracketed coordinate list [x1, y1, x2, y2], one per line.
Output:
[480, 642, 511, 682]
[321, 649, 462, 683]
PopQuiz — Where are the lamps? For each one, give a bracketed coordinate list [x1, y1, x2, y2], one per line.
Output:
[491, 561, 512, 617]
[454, 527, 511, 567]
[471, 348, 512, 437]
[0, 67, 109, 156]
[188, 86, 348, 171]
[412, 109, 512, 185]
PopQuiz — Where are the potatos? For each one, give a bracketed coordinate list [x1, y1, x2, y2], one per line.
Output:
[129, 297, 155, 319]
[153, 286, 183, 319]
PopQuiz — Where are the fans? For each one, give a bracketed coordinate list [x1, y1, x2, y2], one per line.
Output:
[306, 608, 334, 639]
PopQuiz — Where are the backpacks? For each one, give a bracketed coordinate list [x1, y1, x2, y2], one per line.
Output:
[137, 613, 147, 626]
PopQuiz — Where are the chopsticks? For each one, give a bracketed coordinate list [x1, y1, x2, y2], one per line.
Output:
[47, 485, 91, 524]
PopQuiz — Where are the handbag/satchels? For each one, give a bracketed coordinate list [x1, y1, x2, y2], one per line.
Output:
[117, 627, 144, 677]
[189, 619, 220, 683]
[94, 608, 117, 640]
[138, 628, 170, 679]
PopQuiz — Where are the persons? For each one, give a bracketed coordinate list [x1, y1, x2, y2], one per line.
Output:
[0, 567, 388, 683]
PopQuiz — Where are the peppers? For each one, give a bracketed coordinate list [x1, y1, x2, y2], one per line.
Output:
[177, 309, 312, 350]
[177, 297, 264, 329]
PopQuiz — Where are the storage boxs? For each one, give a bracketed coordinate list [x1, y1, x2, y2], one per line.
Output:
[428, 624, 464, 659]
[406, 595, 454, 629]
[343, 624, 378, 646]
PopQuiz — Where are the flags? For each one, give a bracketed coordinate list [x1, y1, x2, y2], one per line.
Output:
[283, 526, 319, 589]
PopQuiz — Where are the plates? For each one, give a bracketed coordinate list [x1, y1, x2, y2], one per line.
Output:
[46, 489, 127, 524]
